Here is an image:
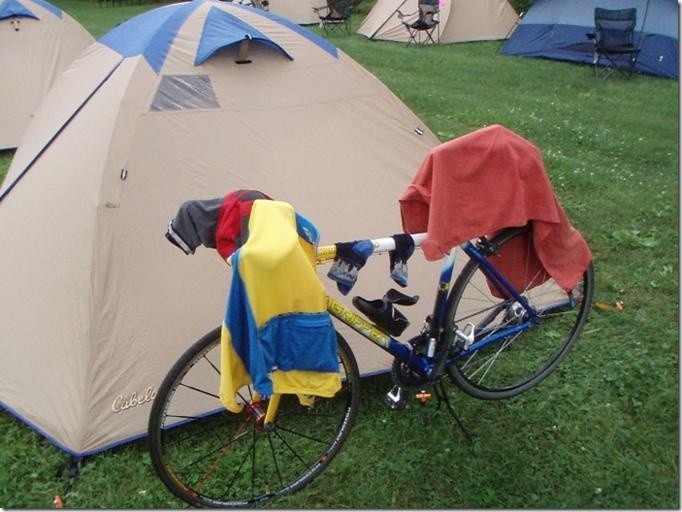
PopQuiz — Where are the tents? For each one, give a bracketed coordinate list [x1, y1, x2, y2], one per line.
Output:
[1, 0, 98, 150]
[497, 1, 680, 84]
[1, 1, 582, 478]
[231, 0, 343, 25]
[354, 0, 521, 45]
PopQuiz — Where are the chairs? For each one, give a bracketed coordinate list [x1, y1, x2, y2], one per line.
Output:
[396, 0, 440, 48]
[585, 7, 656, 83]
[312, 0, 354, 36]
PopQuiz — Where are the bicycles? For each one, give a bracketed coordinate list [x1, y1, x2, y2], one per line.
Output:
[148, 220, 594, 508]
